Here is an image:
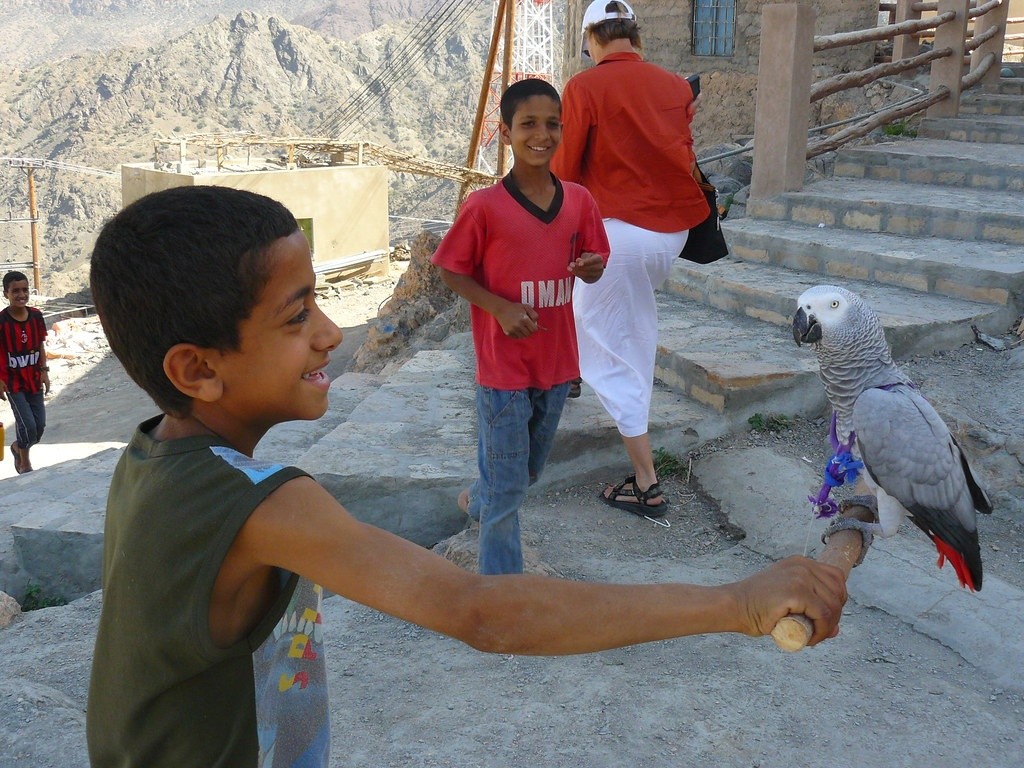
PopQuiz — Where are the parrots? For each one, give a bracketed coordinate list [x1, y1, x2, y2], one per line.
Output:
[792, 285, 993, 594]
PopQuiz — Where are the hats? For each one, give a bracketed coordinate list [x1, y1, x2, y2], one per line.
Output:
[581, 0, 636, 33]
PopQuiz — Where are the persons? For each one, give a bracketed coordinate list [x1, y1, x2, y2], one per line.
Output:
[430, 77, 612, 576]
[87, 182, 848, 767]
[549, 0, 711, 520]
[1, 270, 51, 475]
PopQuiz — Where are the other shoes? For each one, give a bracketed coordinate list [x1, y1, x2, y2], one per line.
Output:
[11, 444, 33, 474]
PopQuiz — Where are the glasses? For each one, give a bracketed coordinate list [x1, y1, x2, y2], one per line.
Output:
[582, 50, 590, 57]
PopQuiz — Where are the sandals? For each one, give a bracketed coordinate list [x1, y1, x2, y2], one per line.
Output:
[600, 476, 667, 518]
[568, 379, 583, 398]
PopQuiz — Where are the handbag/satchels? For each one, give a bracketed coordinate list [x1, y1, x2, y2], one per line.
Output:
[679, 161, 728, 265]
